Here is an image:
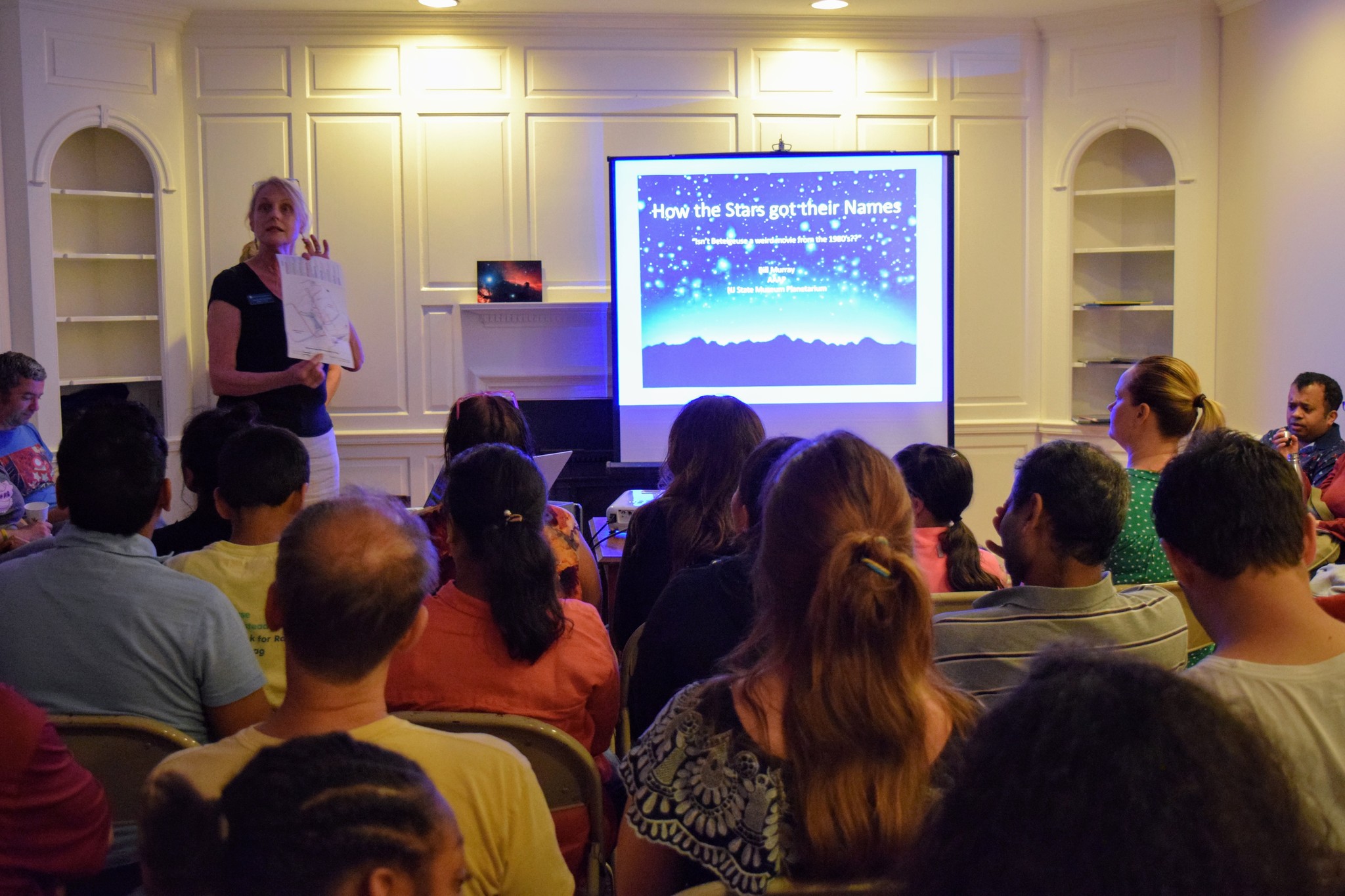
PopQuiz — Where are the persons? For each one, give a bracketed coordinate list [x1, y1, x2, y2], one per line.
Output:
[885, 441, 1011, 594]
[415, 392, 605, 620]
[0, 350, 168, 531]
[154, 488, 577, 896]
[137, 729, 473, 896]
[1098, 354, 1229, 583]
[611, 395, 767, 653]
[1256, 371, 1345, 490]
[1272, 428, 1345, 544]
[608, 429, 993, 896]
[379, 442, 622, 881]
[1, 400, 278, 896]
[902, 641, 1345, 896]
[205, 176, 364, 511]
[617, 433, 811, 758]
[151, 406, 254, 559]
[929, 440, 1190, 724]
[149, 425, 312, 709]
[0, 673, 116, 896]
[0, 461, 54, 554]
[1146, 429, 1345, 896]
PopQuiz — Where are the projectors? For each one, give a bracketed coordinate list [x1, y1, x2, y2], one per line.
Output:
[604, 488, 664, 535]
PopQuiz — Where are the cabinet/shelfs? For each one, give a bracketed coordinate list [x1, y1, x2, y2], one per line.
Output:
[30, 105, 190, 455]
[1036, 107, 1195, 435]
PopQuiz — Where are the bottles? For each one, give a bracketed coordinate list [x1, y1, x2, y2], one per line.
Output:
[1287, 452, 1306, 502]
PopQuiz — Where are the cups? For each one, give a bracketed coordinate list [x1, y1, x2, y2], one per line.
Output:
[24, 501, 50, 524]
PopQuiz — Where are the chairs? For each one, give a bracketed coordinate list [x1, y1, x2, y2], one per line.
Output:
[51, 580, 1214, 896]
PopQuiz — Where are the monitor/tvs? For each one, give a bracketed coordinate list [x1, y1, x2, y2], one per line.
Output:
[477, 260, 542, 303]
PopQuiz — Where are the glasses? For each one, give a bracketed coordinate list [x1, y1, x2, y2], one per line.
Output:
[252, 178, 301, 194]
[456, 390, 519, 420]
